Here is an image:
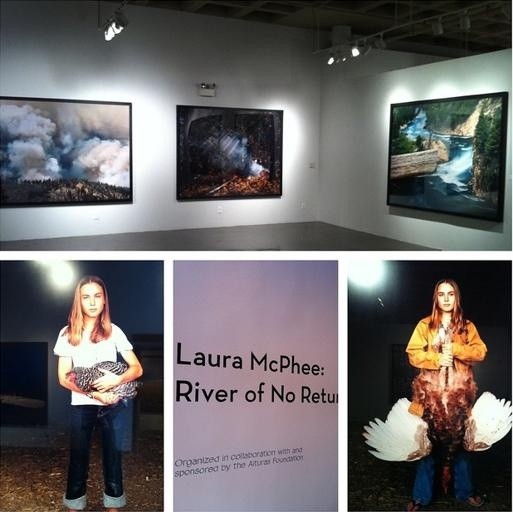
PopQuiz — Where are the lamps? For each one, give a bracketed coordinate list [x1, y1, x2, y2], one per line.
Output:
[312, 0, 512, 65]
[97, 0, 129, 41]
[200, 80, 216, 97]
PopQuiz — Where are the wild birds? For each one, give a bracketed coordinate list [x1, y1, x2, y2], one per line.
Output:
[360, 325, 512, 496]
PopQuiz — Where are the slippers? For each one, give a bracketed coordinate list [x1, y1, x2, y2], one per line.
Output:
[466, 495, 485, 507]
[407, 500, 422, 512]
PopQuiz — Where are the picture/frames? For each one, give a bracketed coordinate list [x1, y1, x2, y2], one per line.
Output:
[0, 96, 132, 209]
[176, 105, 283, 198]
[386, 91, 508, 222]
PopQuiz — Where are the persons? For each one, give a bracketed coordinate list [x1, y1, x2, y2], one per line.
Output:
[52, 274, 145, 512]
[402, 276, 489, 510]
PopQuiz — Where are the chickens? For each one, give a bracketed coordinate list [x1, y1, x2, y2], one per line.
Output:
[67, 361, 144, 401]
